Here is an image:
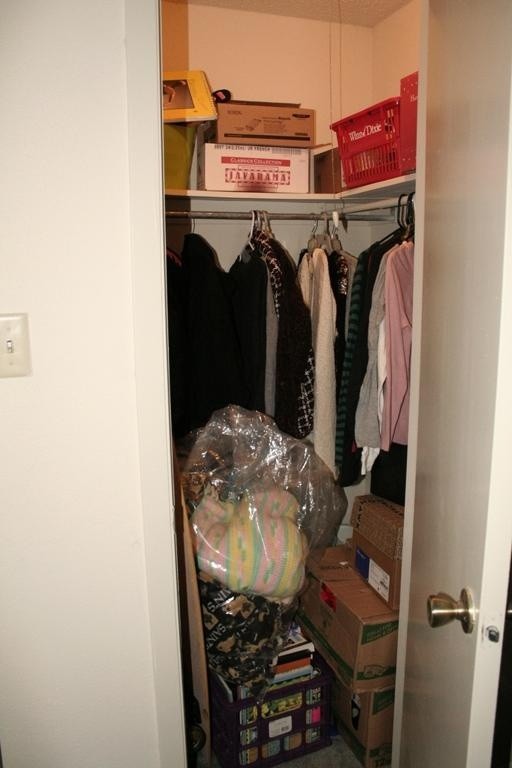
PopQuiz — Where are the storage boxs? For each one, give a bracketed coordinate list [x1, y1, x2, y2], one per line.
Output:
[302, 546, 396, 691]
[344, 533, 400, 613]
[196, 143, 314, 194]
[350, 493, 405, 560]
[207, 652, 337, 767]
[329, 93, 400, 188]
[332, 681, 396, 767]
[206, 101, 317, 149]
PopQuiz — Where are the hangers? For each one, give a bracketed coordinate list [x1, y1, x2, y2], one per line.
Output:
[377, 191, 420, 245]
[239, 208, 341, 253]
